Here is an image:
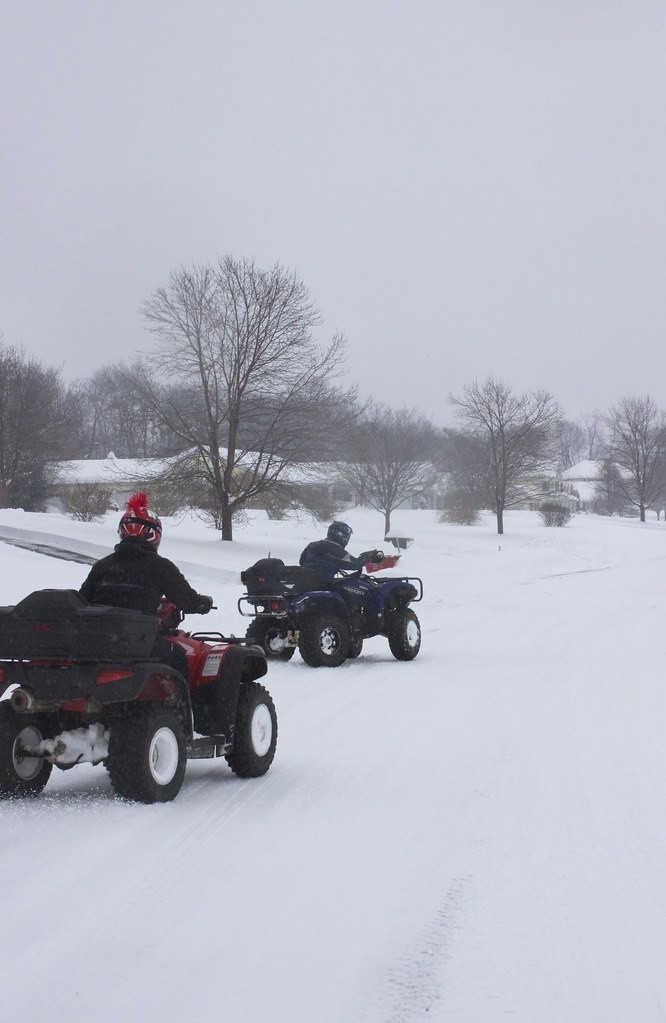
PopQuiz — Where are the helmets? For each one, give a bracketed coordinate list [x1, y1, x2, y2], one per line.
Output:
[326, 521, 352, 548]
[117, 507, 163, 547]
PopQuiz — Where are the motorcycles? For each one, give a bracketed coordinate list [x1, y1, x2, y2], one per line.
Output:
[0, 580, 278, 805]
[238, 552, 423, 667]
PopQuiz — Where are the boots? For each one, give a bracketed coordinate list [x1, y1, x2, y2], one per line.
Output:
[190, 688, 220, 736]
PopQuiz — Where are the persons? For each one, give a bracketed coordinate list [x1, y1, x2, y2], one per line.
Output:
[298, 521, 366, 639]
[79, 494, 214, 684]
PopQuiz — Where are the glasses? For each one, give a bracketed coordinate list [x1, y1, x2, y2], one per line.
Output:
[156, 525, 162, 538]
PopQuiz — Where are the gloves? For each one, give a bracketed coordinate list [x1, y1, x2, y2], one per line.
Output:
[361, 549, 385, 564]
[199, 594, 213, 615]
